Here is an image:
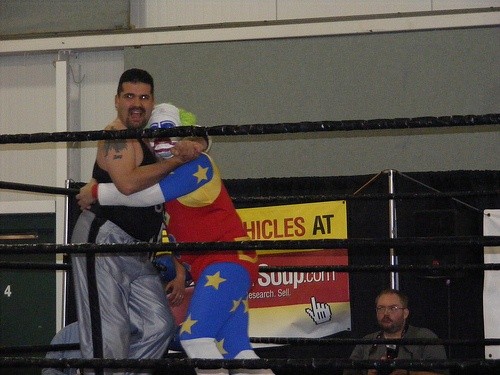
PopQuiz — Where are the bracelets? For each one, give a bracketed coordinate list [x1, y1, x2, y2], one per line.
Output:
[90, 184, 99, 199]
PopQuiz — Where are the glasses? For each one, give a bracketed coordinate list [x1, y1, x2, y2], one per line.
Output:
[377, 305, 406, 313]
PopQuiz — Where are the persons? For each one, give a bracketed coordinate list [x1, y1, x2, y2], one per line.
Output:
[69, 68, 208, 375]
[41, 103, 448, 375]
